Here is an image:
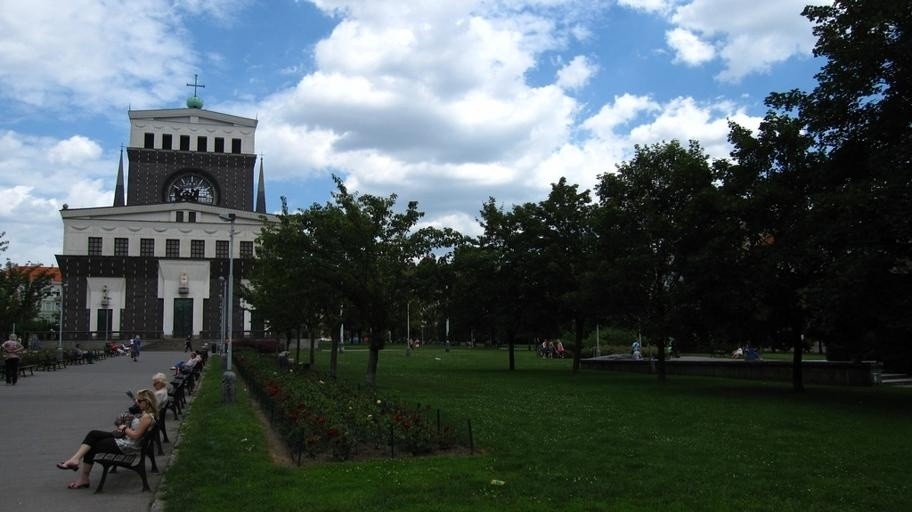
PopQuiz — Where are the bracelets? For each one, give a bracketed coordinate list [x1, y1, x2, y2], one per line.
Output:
[122, 426, 128, 433]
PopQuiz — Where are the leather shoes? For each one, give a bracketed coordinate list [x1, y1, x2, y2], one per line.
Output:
[68, 482, 90, 490]
[56, 461, 80, 472]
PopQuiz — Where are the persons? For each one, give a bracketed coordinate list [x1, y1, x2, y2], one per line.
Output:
[57, 388, 159, 489]
[73, 334, 142, 364]
[166, 334, 211, 412]
[1, 334, 25, 386]
[408, 337, 420, 353]
[542, 336, 760, 365]
[114, 373, 168, 427]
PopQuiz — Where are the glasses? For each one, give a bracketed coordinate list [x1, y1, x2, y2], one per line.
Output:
[137, 399, 149, 403]
[153, 381, 161, 387]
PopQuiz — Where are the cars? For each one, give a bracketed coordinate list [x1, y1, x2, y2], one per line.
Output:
[226, 330, 278, 353]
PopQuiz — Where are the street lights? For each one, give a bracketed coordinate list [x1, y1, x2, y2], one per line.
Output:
[420, 325, 424, 346]
[57, 282, 70, 349]
[100, 284, 111, 341]
[339, 300, 345, 352]
[406, 298, 414, 348]
[218, 214, 235, 393]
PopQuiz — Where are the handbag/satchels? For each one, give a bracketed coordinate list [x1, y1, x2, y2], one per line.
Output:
[111, 431, 124, 439]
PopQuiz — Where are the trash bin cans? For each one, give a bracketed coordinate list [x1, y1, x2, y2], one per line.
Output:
[211, 343, 216, 353]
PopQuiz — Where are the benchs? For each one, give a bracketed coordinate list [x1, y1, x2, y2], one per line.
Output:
[95, 346, 208, 494]
[1, 343, 128, 379]
